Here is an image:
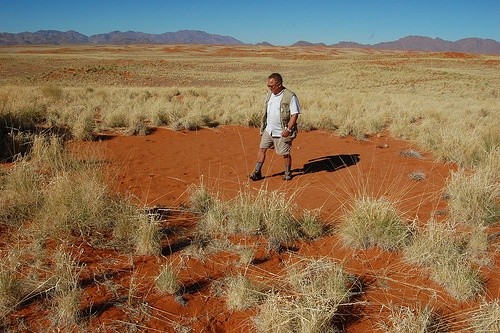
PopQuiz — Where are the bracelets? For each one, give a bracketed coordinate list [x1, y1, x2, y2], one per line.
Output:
[285, 127, 292, 132]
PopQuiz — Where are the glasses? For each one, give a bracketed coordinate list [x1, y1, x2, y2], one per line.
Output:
[267, 83, 280, 88]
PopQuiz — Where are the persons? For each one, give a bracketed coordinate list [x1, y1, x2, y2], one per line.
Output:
[246, 72, 301, 182]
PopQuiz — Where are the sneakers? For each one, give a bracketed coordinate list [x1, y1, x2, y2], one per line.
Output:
[249, 171, 262, 180]
[282, 171, 292, 179]
